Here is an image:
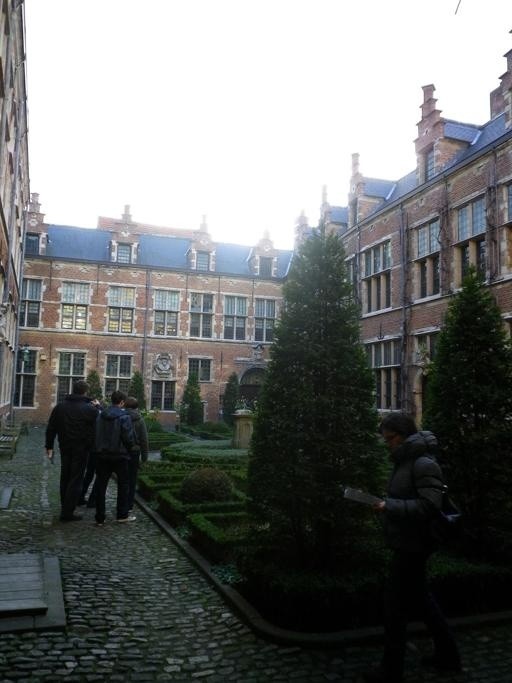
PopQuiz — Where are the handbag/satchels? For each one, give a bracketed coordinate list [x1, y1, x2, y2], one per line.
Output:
[426, 488, 461, 547]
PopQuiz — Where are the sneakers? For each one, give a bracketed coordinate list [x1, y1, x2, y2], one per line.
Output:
[361, 666, 404, 683]
[77, 498, 97, 508]
[420, 655, 464, 673]
[95, 515, 137, 526]
[59, 510, 84, 525]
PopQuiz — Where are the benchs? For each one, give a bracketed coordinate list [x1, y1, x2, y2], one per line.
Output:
[1, 417, 25, 460]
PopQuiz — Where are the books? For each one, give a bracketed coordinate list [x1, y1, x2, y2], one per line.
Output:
[343, 487, 376, 505]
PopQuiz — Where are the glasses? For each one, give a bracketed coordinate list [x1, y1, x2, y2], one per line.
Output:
[383, 432, 399, 444]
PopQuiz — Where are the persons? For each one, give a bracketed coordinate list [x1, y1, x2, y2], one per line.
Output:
[362, 412, 468, 681]
[45, 381, 149, 526]
